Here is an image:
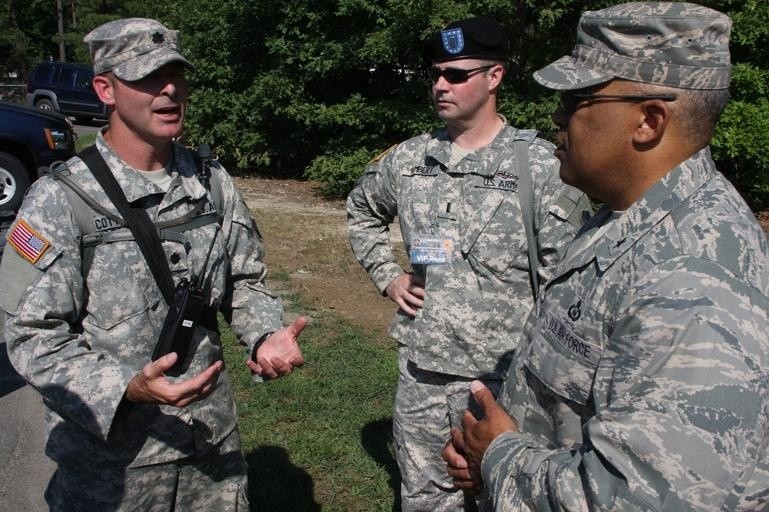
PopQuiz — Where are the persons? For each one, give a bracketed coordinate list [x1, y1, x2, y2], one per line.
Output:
[343, 15, 597, 510]
[1, 16, 308, 510]
[440, 1, 769, 511]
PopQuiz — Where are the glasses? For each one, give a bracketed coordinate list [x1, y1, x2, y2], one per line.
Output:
[426, 64, 498, 84]
[560, 91, 676, 117]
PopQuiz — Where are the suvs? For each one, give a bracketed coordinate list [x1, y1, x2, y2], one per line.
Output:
[0, 99, 77, 223]
[23, 57, 111, 122]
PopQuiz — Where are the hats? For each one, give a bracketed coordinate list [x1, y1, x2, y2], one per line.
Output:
[533, 1, 733, 91]
[83, 18, 196, 83]
[421, 17, 511, 64]
